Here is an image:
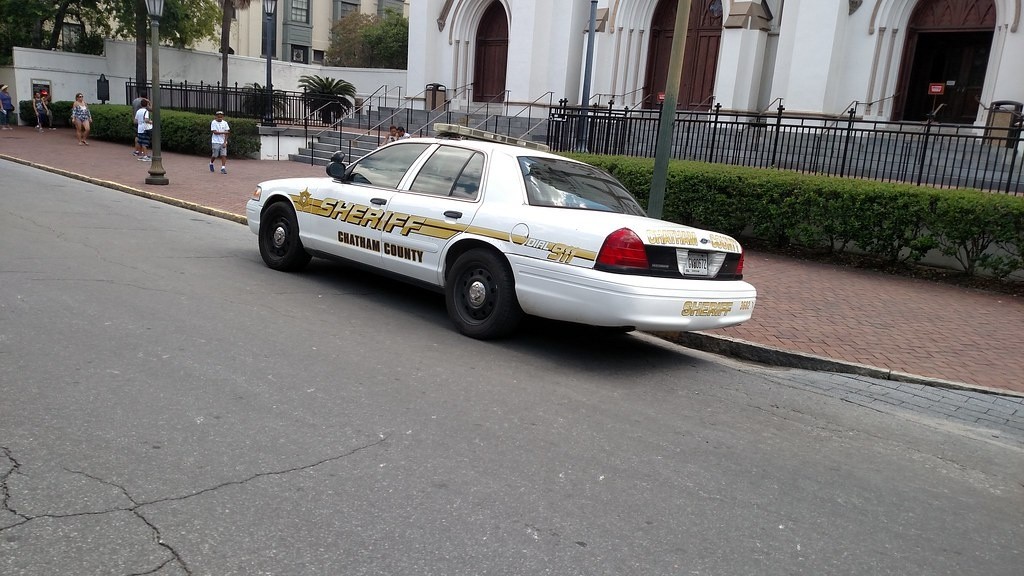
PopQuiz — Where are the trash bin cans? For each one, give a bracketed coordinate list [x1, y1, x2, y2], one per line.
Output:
[982, 100, 1024, 148]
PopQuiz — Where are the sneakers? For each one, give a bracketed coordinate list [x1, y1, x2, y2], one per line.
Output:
[209, 161, 214, 173]
[221, 167, 227, 174]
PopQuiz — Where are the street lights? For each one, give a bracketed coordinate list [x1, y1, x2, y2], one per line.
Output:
[144, 0, 170, 187]
[261, 0, 277, 126]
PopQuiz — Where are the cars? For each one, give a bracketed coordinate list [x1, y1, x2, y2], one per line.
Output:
[246, 123, 757, 341]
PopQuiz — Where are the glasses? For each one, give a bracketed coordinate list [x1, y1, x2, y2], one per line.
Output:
[215, 111, 225, 115]
[79, 96, 83, 98]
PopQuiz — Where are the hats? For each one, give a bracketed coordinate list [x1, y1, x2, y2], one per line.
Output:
[1, 85, 8, 91]
[41, 90, 47, 95]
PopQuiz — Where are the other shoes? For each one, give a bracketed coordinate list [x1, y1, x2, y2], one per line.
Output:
[2, 127, 13, 130]
[35, 125, 44, 133]
[48, 128, 56, 131]
[79, 140, 88, 146]
[133, 151, 152, 162]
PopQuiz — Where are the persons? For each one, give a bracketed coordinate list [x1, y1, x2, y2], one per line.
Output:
[0, 84, 15, 130]
[70, 93, 92, 146]
[130, 90, 153, 162]
[32, 90, 57, 132]
[380, 126, 412, 146]
[208, 111, 229, 173]
